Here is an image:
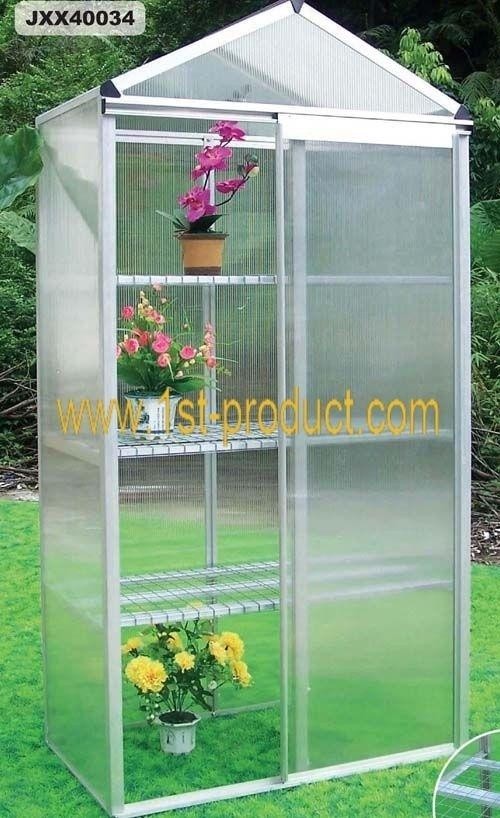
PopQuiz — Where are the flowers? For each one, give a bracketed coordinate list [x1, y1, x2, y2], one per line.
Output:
[120, 602, 256, 722]
[114, 282, 244, 401]
[155, 117, 261, 233]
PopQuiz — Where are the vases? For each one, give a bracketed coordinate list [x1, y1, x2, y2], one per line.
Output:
[175, 233, 228, 276]
[125, 389, 182, 442]
[158, 711, 199, 756]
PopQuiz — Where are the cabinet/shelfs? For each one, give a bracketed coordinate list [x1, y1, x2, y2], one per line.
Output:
[31, 0, 474, 818]
